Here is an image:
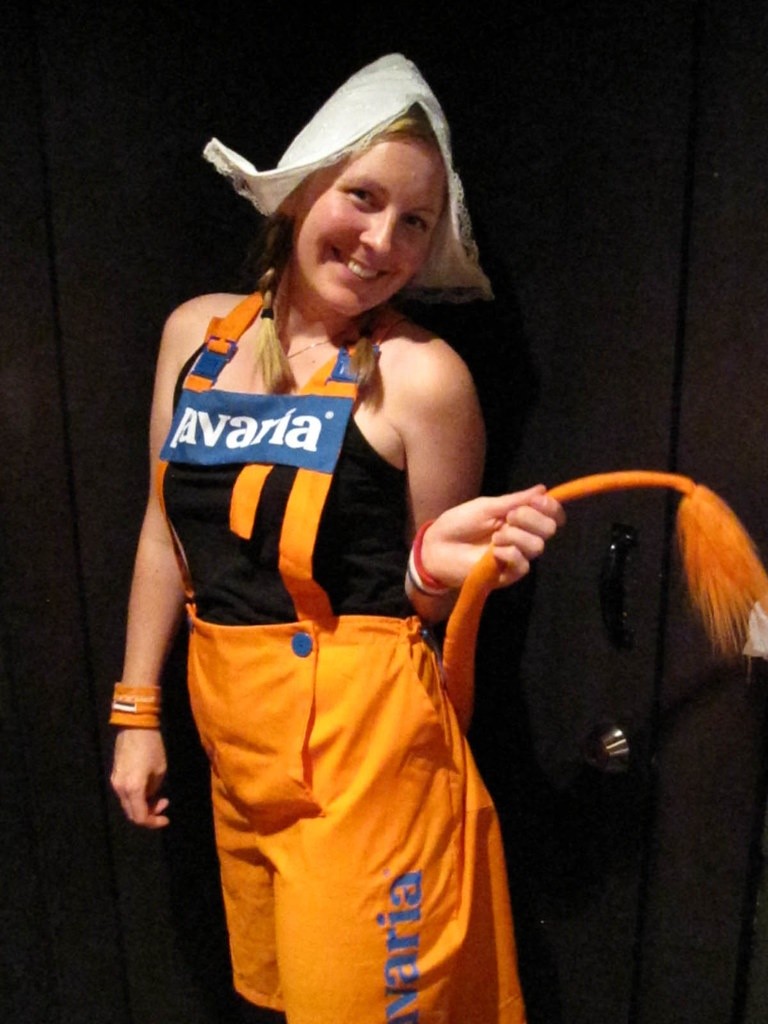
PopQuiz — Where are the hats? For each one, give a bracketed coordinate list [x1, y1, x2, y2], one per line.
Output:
[202, 54, 495, 302]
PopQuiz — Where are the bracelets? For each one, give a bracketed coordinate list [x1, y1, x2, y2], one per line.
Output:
[408, 520, 452, 598]
[109, 682, 162, 730]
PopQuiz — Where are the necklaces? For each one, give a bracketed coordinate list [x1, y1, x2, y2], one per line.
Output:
[286, 319, 358, 359]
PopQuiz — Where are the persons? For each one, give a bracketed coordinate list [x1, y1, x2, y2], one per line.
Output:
[108, 53, 564, 1024]
